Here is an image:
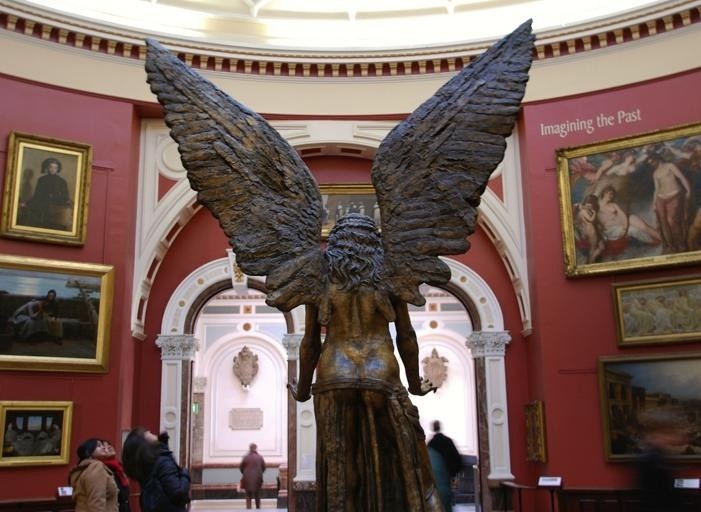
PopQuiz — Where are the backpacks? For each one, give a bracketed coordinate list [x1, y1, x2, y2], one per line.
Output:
[139, 453, 183, 512]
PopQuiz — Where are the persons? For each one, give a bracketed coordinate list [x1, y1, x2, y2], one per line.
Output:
[239, 444, 267, 509]
[121, 426, 191, 512]
[424, 421, 462, 512]
[99, 439, 133, 512]
[10, 297, 50, 344]
[69, 438, 120, 512]
[622, 288, 696, 338]
[29, 288, 64, 346]
[573, 135, 700, 265]
[286, 212, 448, 512]
[16, 158, 73, 232]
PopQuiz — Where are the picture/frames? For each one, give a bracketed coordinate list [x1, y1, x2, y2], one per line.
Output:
[554, 121, 700, 280]
[523, 399, 548, 463]
[311, 182, 383, 241]
[0, 129, 114, 469]
[611, 274, 701, 348]
[597, 350, 701, 465]
